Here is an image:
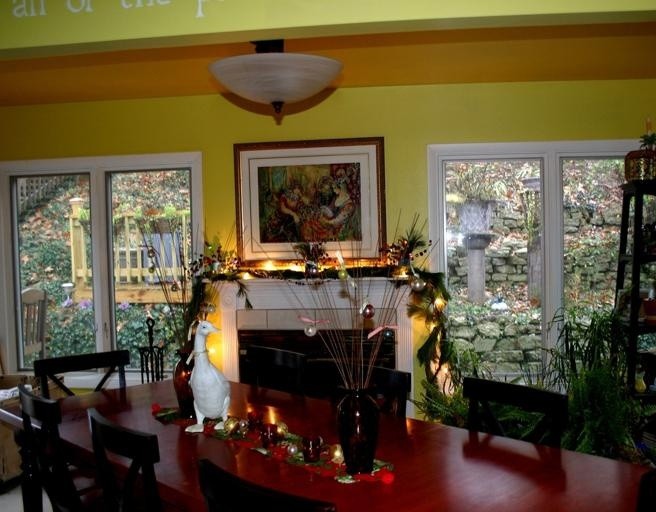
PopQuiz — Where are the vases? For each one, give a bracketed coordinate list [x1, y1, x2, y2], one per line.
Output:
[624, 150, 656, 181]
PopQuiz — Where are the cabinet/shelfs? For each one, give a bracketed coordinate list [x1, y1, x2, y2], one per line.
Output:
[613, 181, 656, 402]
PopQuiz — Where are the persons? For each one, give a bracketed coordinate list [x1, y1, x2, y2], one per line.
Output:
[265, 168, 361, 242]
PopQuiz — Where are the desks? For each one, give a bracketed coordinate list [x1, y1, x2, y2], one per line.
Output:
[1, 377, 655, 510]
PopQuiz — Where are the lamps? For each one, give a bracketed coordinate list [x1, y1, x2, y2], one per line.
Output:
[209, 38, 344, 114]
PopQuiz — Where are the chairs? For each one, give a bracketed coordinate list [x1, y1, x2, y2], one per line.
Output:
[34, 349, 130, 398]
[247, 347, 306, 393]
[21, 288, 47, 361]
[16, 381, 101, 511]
[197, 459, 334, 510]
[85, 409, 161, 510]
[464, 376, 569, 446]
[307, 360, 412, 416]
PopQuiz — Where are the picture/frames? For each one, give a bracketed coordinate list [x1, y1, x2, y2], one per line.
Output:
[232, 135, 388, 265]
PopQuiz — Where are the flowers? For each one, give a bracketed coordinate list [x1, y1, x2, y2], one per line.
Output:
[639, 116, 655, 150]
